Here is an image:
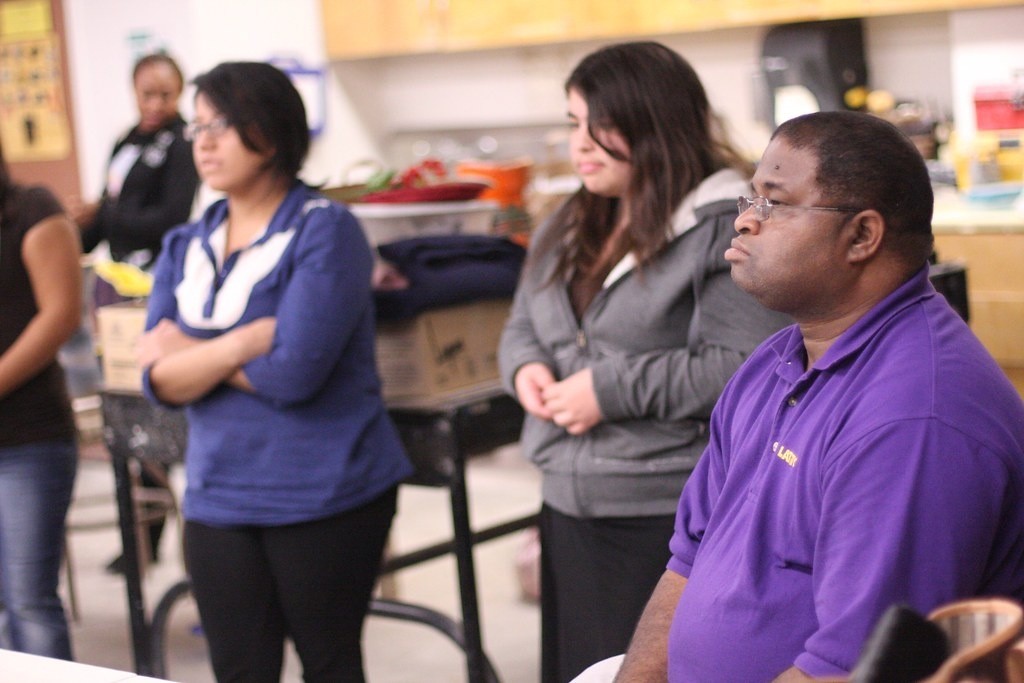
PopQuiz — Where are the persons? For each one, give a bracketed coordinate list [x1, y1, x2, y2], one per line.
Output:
[495, 40, 799, 683]
[68, 48, 203, 574]
[133, 62, 414, 683]
[614, 111, 1024, 682]
[0, 135, 83, 662]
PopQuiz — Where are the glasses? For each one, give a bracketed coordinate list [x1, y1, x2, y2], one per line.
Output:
[736, 196, 864, 222]
[183, 118, 234, 141]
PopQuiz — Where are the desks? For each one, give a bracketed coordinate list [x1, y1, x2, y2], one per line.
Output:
[97, 379, 528, 683]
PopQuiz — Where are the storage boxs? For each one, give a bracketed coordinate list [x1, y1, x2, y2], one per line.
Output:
[373, 302, 518, 396]
[99, 300, 154, 390]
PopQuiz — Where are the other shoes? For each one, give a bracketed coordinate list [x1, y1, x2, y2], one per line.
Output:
[108, 551, 159, 574]
